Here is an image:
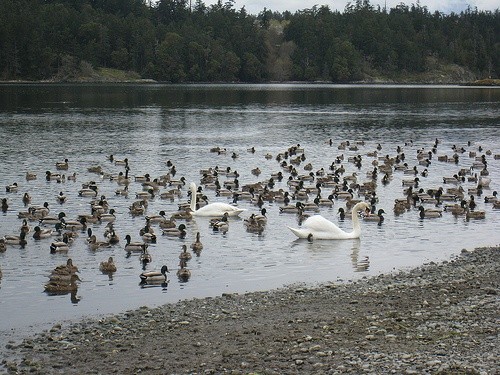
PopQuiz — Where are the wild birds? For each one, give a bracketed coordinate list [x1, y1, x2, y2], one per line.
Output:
[0, 136, 500, 291]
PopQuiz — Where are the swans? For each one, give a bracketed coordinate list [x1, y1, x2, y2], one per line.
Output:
[189, 183, 246, 217]
[287, 202, 371, 240]
